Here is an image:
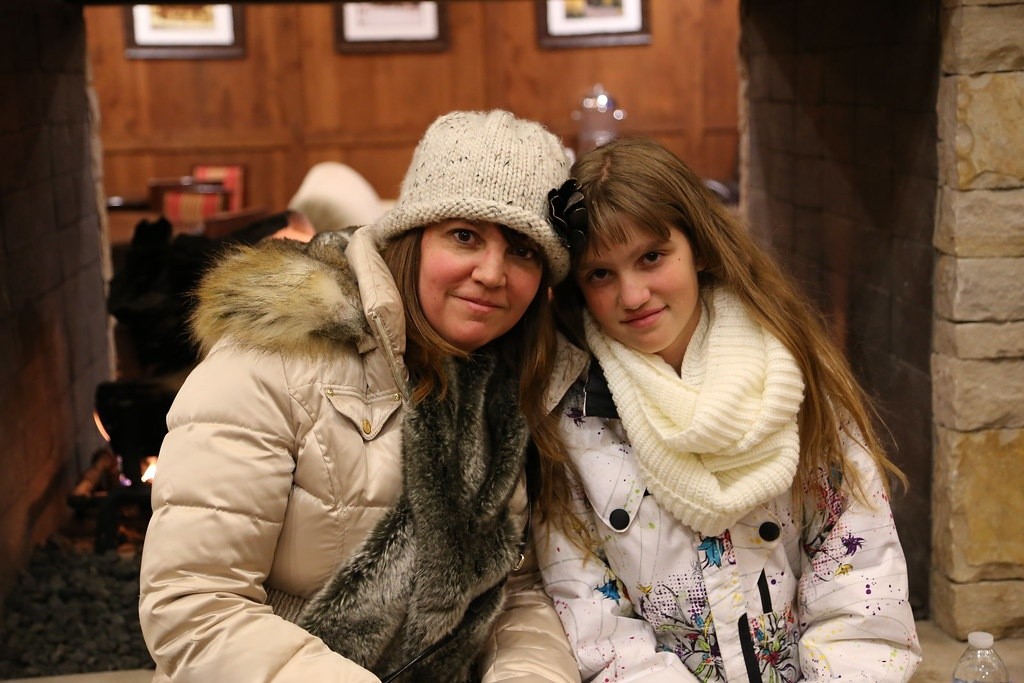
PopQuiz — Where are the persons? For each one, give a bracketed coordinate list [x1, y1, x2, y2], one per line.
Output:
[138, 109, 581, 683]
[533, 134, 923, 683]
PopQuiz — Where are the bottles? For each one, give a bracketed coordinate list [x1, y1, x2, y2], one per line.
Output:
[952, 632, 1009, 683]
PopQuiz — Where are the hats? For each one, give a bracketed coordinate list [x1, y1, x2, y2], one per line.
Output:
[372, 108, 588, 289]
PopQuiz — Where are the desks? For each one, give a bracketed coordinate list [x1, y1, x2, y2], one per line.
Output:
[110, 207, 288, 248]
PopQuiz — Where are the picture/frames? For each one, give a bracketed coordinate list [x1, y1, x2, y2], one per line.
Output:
[332, 0, 448, 55]
[121, 3, 246, 60]
[535, 0, 652, 48]
